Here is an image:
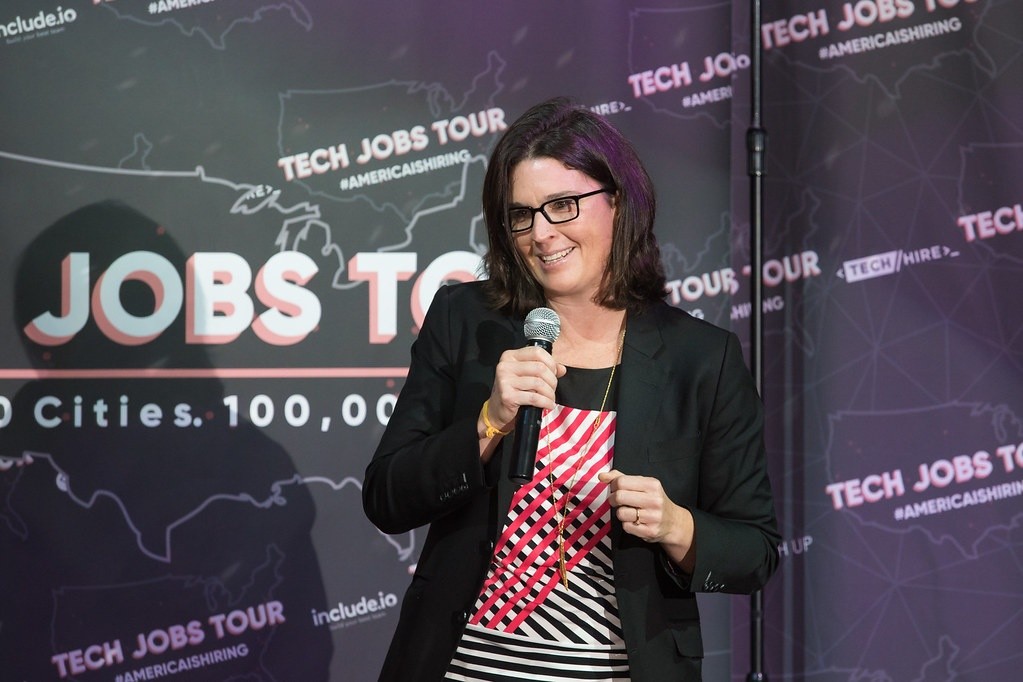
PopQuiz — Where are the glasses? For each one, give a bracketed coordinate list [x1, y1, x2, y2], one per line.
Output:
[502, 187, 610, 232]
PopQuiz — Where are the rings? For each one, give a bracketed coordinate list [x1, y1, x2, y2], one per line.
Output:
[633, 509, 640, 525]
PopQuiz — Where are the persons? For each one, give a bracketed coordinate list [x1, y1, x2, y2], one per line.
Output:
[359, 96, 779, 682]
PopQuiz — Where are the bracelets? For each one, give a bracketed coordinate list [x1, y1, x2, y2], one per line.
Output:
[482, 400, 512, 437]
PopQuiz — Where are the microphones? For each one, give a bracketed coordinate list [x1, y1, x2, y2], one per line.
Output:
[509, 307, 561, 486]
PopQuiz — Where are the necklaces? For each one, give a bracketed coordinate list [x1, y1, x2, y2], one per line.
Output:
[547, 328, 624, 592]
